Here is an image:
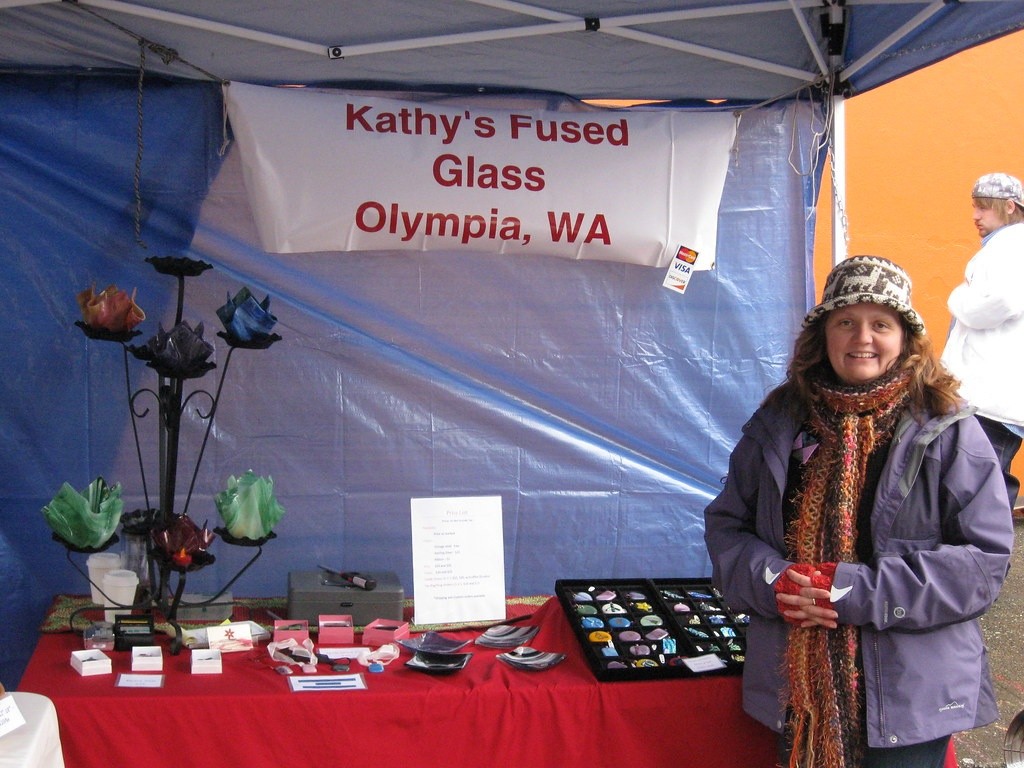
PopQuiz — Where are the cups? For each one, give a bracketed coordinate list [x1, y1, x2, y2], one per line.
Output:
[102, 570, 139, 623]
[123, 532, 148, 587]
[86, 553, 121, 604]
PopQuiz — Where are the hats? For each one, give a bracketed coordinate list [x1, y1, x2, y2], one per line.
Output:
[804, 256, 926, 337]
[971, 173, 1024, 207]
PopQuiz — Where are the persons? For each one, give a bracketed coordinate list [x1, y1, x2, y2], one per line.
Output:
[940, 171, 1024, 513]
[704, 253, 1013, 768]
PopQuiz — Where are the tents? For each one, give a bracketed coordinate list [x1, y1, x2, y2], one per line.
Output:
[0, 0, 1024, 693]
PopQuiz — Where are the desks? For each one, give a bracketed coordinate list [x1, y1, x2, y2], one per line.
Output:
[16, 594, 958, 768]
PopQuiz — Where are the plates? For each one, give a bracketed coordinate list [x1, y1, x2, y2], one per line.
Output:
[405, 652, 473, 676]
[475, 626, 540, 646]
[497, 646, 567, 669]
[395, 633, 473, 652]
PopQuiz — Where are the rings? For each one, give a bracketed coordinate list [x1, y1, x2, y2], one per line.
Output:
[812, 598, 816, 605]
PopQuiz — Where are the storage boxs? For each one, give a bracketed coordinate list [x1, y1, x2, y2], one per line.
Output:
[318, 614, 354, 644]
[131, 646, 163, 671]
[70, 649, 112, 676]
[362, 619, 410, 645]
[287, 571, 405, 626]
[190, 649, 222, 674]
[274, 619, 309, 645]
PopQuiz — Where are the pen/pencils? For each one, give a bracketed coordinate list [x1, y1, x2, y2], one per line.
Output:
[265, 608, 283, 620]
